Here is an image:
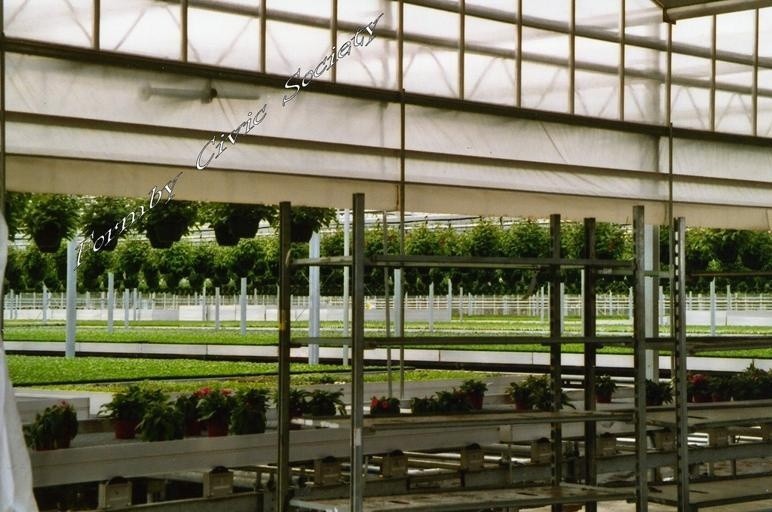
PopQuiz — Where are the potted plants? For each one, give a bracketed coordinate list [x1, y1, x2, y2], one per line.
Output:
[23, 400, 78, 451]
[595, 374, 618, 403]
[134, 387, 346, 442]
[646, 359, 772, 405]
[410, 373, 576, 410]
[96, 387, 149, 439]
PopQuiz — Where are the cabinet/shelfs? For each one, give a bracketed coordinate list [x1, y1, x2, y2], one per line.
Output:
[277, 194, 647, 512]
[585, 217, 772, 511]
